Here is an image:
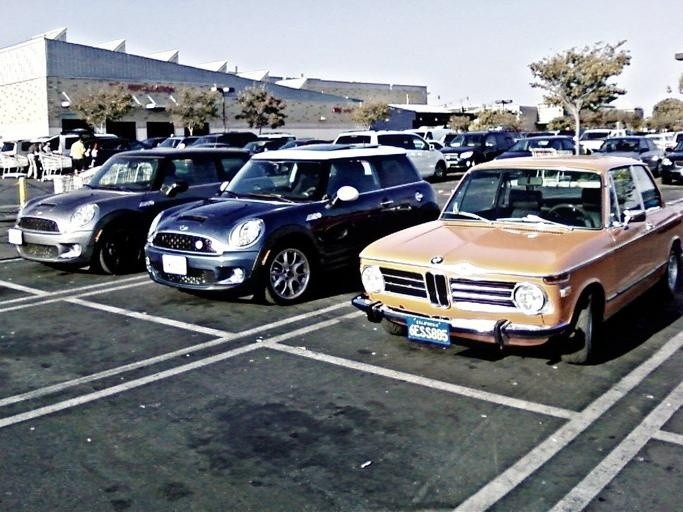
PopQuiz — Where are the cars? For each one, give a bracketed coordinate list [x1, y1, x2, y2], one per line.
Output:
[137, 131, 296, 153]
[496, 128, 683, 184]
[350, 154, 681, 366]
[8, 147, 251, 274]
[142, 144, 441, 306]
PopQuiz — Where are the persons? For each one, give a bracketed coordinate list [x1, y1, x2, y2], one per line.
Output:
[24, 141, 55, 181]
[68, 136, 103, 173]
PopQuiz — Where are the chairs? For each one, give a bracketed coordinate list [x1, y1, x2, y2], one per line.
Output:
[291, 162, 374, 201]
[494, 188, 623, 229]
[151, 160, 223, 188]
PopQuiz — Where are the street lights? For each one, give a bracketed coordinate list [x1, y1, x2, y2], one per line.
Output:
[222, 85, 230, 128]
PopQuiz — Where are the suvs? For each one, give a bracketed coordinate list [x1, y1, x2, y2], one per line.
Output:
[332, 126, 513, 183]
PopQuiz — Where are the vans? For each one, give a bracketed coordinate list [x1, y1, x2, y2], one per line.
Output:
[0, 133, 147, 173]
